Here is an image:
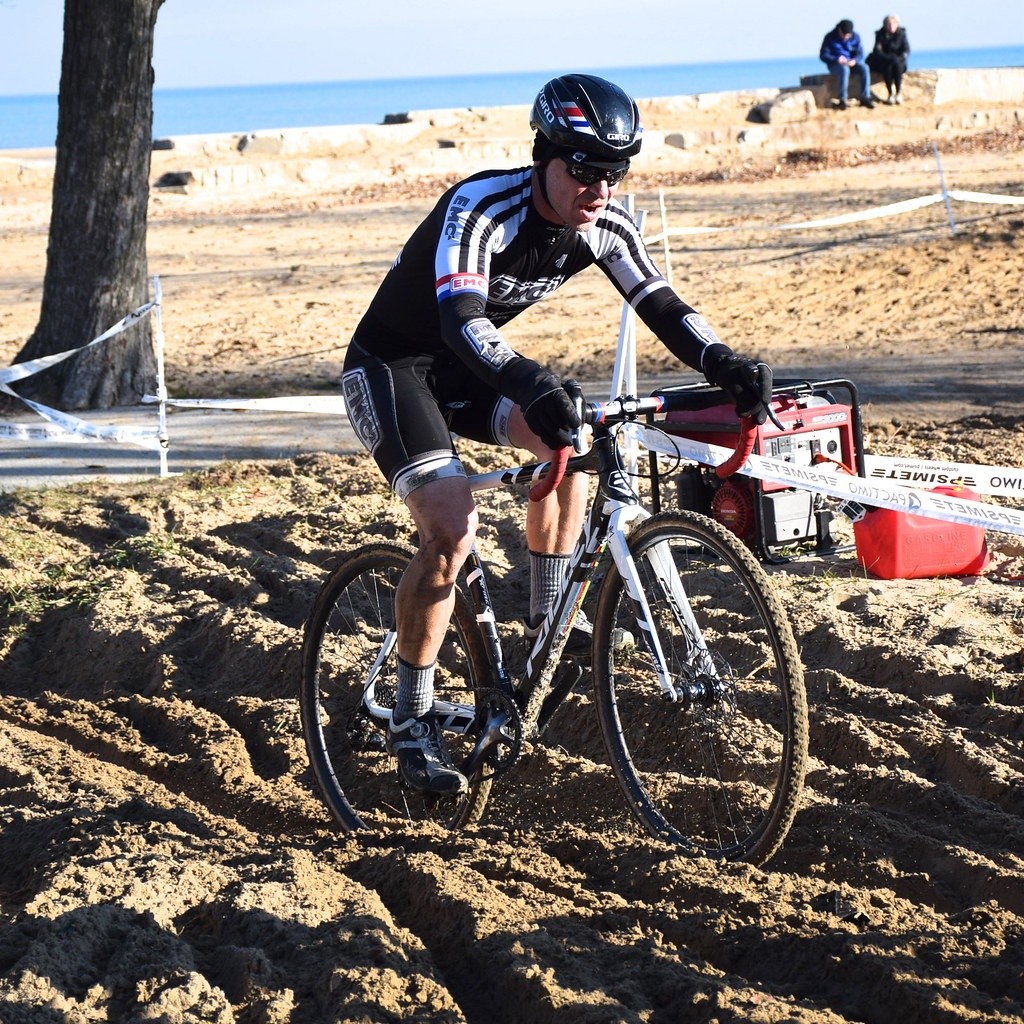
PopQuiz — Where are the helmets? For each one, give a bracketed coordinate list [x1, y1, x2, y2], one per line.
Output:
[530, 74, 643, 157]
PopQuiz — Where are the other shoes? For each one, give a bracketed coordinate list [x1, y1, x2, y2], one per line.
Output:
[838, 93, 903, 110]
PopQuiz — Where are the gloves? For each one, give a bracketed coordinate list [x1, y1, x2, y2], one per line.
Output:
[703, 344, 772, 424]
[519, 372, 581, 450]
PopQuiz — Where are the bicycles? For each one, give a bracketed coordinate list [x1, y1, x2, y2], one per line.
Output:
[299, 358, 809, 870]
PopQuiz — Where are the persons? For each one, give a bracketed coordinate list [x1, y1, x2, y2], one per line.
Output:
[820, 20, 876, 108]
[873, 16, 909, 104]
[343, 75, 770, 795]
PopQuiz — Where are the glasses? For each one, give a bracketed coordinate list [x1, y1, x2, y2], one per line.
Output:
[556, 151, 630, 187]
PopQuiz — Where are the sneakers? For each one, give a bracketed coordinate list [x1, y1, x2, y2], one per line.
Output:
[521, 609, 634, 660]
[386, 701, 468, 794]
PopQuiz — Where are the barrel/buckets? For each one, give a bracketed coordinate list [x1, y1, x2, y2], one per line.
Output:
[854, 485, 990, 579]
